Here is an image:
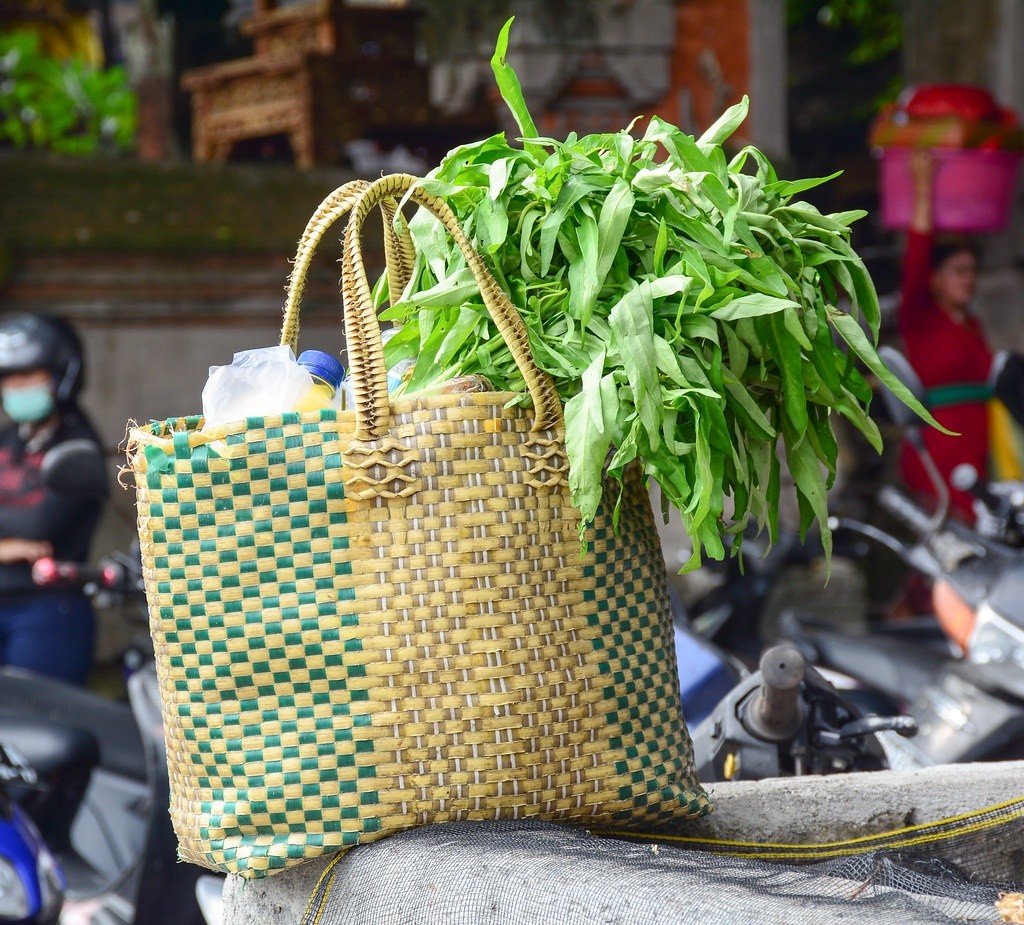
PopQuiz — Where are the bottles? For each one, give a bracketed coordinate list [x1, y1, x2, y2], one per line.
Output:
[296, 348, 344, 413]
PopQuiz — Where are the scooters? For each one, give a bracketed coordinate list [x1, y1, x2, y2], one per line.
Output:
[645, 342, 1022, 780]
[0, 537, 200, 924]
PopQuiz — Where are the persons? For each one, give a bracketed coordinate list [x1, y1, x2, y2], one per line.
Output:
[897, 146, 996, 617]
[0, 310, 108, 688]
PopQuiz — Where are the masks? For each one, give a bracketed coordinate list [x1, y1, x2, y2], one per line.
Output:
[2, 384, 59, 421]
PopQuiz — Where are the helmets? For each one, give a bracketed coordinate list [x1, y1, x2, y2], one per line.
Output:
[0, 310, 84, 403]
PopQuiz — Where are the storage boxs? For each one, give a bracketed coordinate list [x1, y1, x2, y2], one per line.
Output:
[880, 146, 1024, 233]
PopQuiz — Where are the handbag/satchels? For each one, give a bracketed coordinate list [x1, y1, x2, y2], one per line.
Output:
[125, 174, 720, 869]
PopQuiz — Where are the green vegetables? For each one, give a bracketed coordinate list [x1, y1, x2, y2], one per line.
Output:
[369, 14, 961, 592]
